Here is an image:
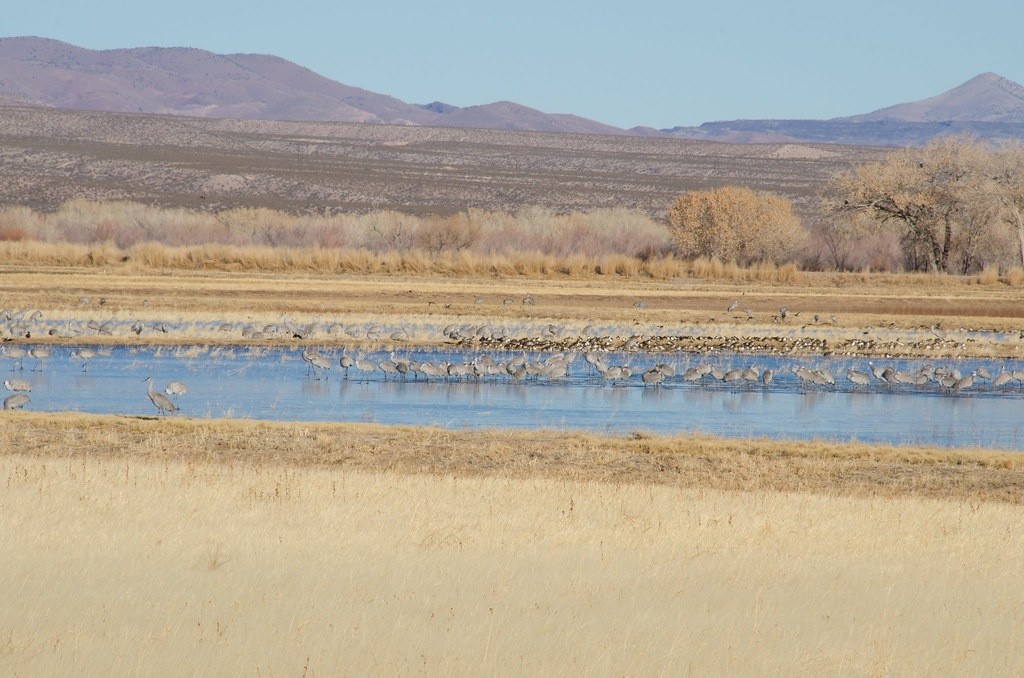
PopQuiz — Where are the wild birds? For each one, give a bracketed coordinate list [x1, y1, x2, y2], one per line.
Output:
[142, 377, 176, 421]
[0, 299, 1024, 396]
[3, 394, 30, 410]
